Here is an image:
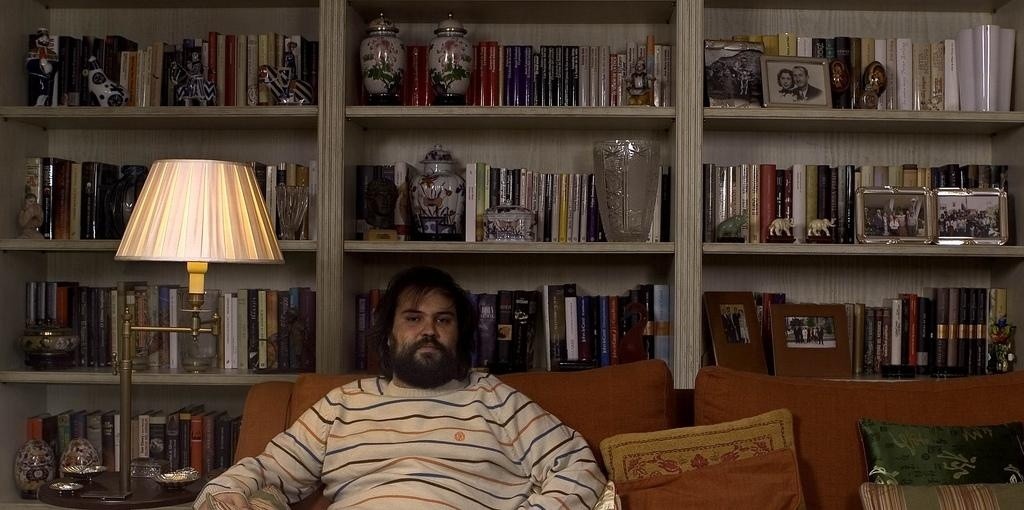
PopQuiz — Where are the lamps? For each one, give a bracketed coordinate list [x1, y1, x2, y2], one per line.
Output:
[81, 157, 283, 500]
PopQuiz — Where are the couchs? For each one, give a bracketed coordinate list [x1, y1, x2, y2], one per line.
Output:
[206, 359, 1024, 510]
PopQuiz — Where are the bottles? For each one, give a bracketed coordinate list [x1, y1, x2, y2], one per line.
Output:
[592, 138, 661, 242]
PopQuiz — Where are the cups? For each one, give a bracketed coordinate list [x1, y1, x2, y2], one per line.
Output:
[276, 182, 311, 241]
[176, 286, 220, 374]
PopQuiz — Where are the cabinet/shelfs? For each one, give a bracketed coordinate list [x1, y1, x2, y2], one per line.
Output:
[1, 0, 331, 384]
[331, 0, 705, 392]
[676, 0, 1024, 389]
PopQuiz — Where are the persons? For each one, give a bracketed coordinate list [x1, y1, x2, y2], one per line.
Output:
[794, 325, 825, 343]
[869, 205, 924, 235]
[776, 69, 794, 96]
[194, 271, 606, 509]
[940, 207, 1000, 236]
[722, 307, 750, 343]
[792, 66, 822, 102]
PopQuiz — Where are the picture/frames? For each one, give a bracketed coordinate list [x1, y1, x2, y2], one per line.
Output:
[769, 303, 852, 379]
[703, 290, 766, 374]
[856, 186, 932, 244]
[705, 39, 766, 108]
[932, 187, 1011, 244]
[759, 54, 831, 110]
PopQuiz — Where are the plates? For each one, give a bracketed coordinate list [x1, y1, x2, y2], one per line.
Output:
[48, 481, 85, 494]
[152, 467, 201, 491]
[59, 465, 111, 481]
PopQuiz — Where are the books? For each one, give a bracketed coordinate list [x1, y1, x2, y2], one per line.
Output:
[827, 24, 1018, 109]
[26, 32, 318, 108]
[855, 164, 1010, 188]
[846, 287, 1008, 376]
[757, 291, 785, 342]
[24, 280, 315, 373]
[29, 404, 242, 474]
[733, 34, 826, 58]
[25, 154, 308, 241]
[403, 35, 671, 105]
[703, 163, 855, 244]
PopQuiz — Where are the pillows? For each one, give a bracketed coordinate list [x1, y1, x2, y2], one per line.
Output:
[613, 448, 806, 510]
[860, 482, 1024, 510]
[856, 416, 1024, 484]
[600, 408, 793, 482]
